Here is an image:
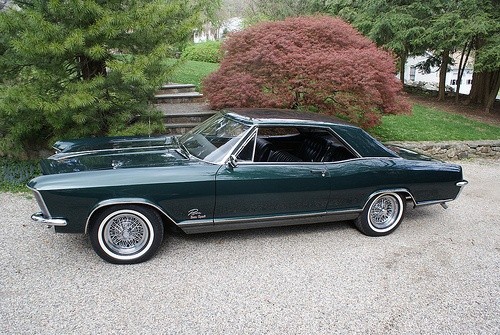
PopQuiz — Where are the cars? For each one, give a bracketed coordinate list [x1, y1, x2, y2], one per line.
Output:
[24, 107, 468, 264]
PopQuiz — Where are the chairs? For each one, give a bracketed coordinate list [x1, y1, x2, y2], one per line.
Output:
[240, 138, 271, 162]
[266, 150, 300, 163]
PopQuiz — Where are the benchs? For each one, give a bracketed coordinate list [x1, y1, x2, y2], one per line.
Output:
[297, 132, 350, 163]
